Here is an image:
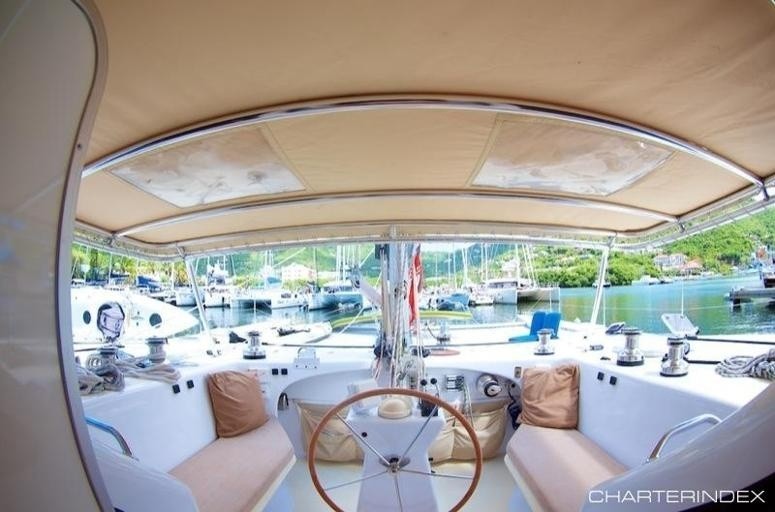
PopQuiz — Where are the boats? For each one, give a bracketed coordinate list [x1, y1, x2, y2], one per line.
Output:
[661, 278, 674, 284]
[592, 279, 609, 288]
[761, 264, 775, 289]
[630, 273, 662, 284]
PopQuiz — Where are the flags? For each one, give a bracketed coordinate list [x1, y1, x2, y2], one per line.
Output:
[404, 255, 423, 324]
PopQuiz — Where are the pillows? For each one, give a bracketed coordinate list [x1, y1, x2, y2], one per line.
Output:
[207, 370, 270, 437]
[516, 362, 580, 428]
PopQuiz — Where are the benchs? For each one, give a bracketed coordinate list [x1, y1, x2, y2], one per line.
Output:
[82, 365, 297, 512]
[504, 358, 722, 512]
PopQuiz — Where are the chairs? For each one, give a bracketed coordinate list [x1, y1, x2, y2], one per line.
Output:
[509, 312, 561, 342]
